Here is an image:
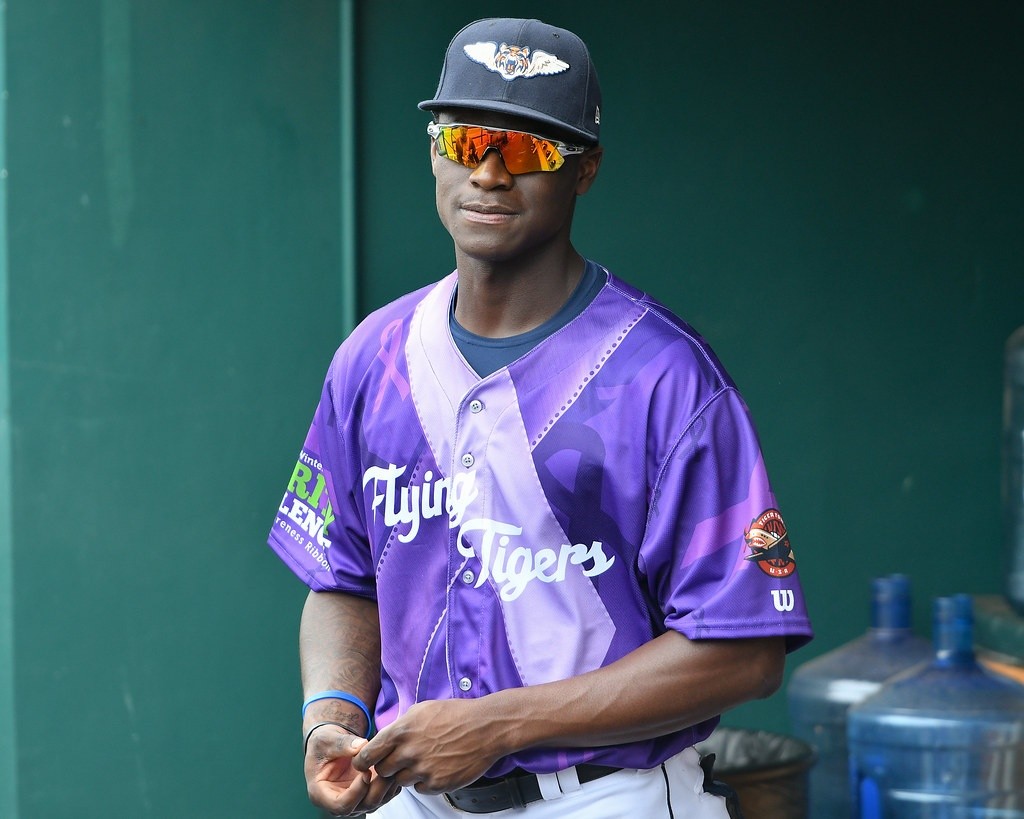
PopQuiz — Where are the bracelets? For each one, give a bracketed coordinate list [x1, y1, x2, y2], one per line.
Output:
[304, 722, 361, 755]
[302, 690, 371, 740]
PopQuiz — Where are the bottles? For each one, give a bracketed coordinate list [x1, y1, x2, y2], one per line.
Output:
[853, 595, 1024, 819]
[784, 576, 926, 819]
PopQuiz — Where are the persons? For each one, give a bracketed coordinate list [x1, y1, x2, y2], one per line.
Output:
[266, 20, 812, 819]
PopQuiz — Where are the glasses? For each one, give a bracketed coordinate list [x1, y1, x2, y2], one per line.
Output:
[427, 121, 589, 174]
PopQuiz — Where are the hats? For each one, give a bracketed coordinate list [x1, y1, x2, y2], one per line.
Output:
[418, 17, 601, 143]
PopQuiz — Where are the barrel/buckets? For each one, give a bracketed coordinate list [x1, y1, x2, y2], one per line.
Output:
[703, 727, 816, 819]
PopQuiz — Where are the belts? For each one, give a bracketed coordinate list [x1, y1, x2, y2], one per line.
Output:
[443, 761, 622, 814]
